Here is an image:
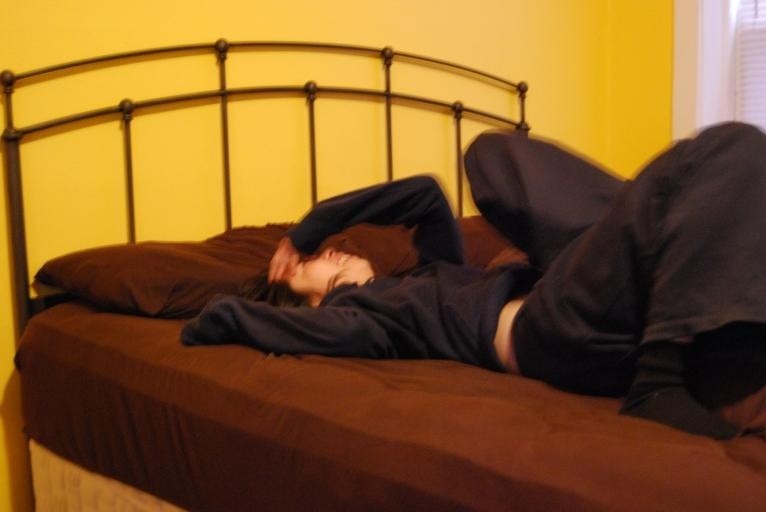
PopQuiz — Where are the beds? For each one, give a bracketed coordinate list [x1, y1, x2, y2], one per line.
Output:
[0, 39, 766, 510]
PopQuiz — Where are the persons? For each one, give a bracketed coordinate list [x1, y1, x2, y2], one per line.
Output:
[179, 123, 766, 442]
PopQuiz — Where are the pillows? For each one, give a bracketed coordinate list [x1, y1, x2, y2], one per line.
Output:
[292, 214, 511, 277]
[35, 225, 288, 320]
